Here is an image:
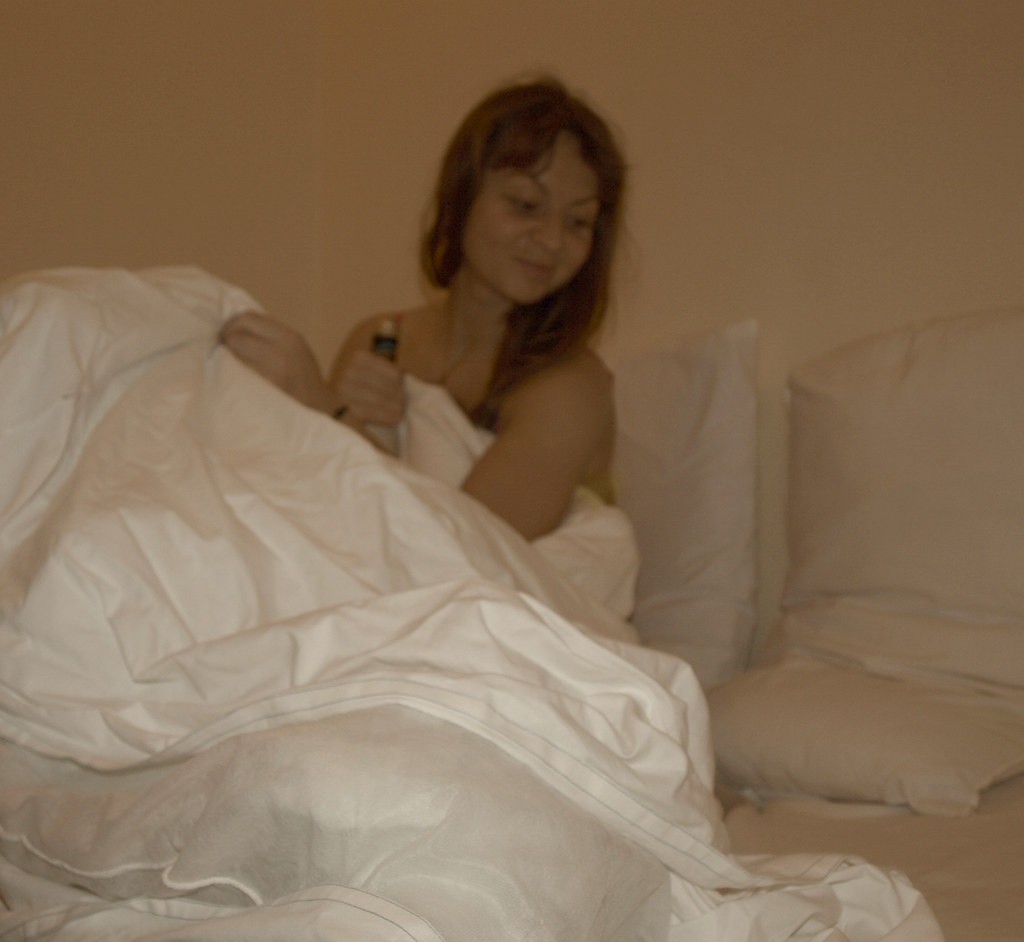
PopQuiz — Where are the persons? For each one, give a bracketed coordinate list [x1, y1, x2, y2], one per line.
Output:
[217, 79, 620, 546]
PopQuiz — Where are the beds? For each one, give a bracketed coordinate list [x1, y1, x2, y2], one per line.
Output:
[0, 264, 1024, 942]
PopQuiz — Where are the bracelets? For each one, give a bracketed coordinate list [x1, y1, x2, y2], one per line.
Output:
[335, 406, 349, 418]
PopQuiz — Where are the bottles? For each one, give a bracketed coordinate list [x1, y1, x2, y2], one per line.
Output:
[332, 319, 401, 464]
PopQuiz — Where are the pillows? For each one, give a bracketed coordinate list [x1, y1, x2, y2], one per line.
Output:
[592, 315, 759, 697]
[705, 305, 1024, 821]
